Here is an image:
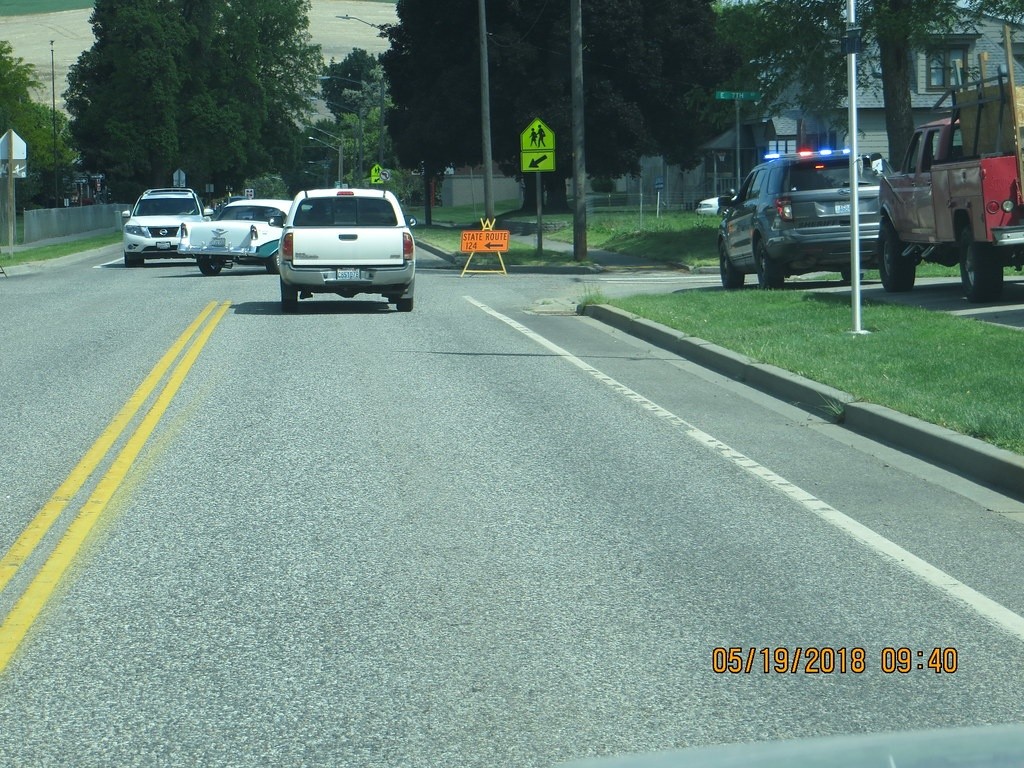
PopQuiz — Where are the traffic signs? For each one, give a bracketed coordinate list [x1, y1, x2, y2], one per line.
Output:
[459, 230, 510, 252]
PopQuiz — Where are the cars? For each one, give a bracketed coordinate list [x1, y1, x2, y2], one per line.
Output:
[279, 187, 415, 312]
[695, 196, 720, 216]
[189, 196, 293, 277]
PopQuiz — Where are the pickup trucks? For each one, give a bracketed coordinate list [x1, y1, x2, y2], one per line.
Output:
[870, 119, 1024, 305]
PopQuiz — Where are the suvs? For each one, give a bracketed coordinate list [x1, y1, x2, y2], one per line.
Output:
[122, 187, 214, 267]
[716, 150, 885, 290]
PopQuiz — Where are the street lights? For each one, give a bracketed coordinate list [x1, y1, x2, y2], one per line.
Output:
[305, 124, 342, 142]
[310, 97, 363, 187]
[316, 74, 385, 166]
[308, 137, 344, 189]
